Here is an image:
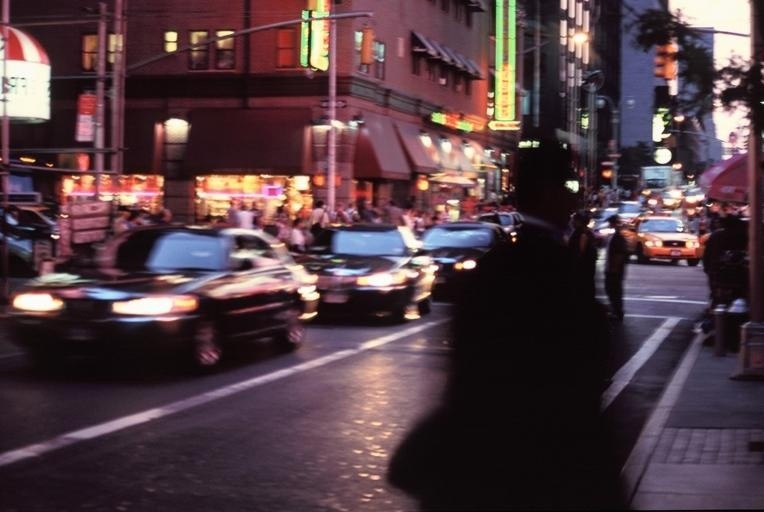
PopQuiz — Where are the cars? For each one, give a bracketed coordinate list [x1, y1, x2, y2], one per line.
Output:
[587, 186, 702, 265]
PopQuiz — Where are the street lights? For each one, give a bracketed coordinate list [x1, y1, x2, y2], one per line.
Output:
[595, 94, 635, 196]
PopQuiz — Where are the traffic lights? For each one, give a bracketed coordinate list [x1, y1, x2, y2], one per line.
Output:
[654, 36, 680, 81]
[361, 27, 377, 66]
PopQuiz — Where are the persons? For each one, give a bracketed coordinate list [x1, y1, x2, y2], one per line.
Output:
[387, 116, 632, 512]
[602, 215, 631, 320]
[1, 203, 21, 241]
[202, 199, 361, 255]
[565, 209, 598, 319]
[109, 198, 172, 237]
[578, 183, 639, 208]
[362, 196, 513, 236]
[695, 203, 752, 344]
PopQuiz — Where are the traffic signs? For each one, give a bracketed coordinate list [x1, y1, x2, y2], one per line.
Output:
[319, 100, 347, 110]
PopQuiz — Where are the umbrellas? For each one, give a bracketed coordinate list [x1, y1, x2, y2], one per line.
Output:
[696, 152, 751, 203]
